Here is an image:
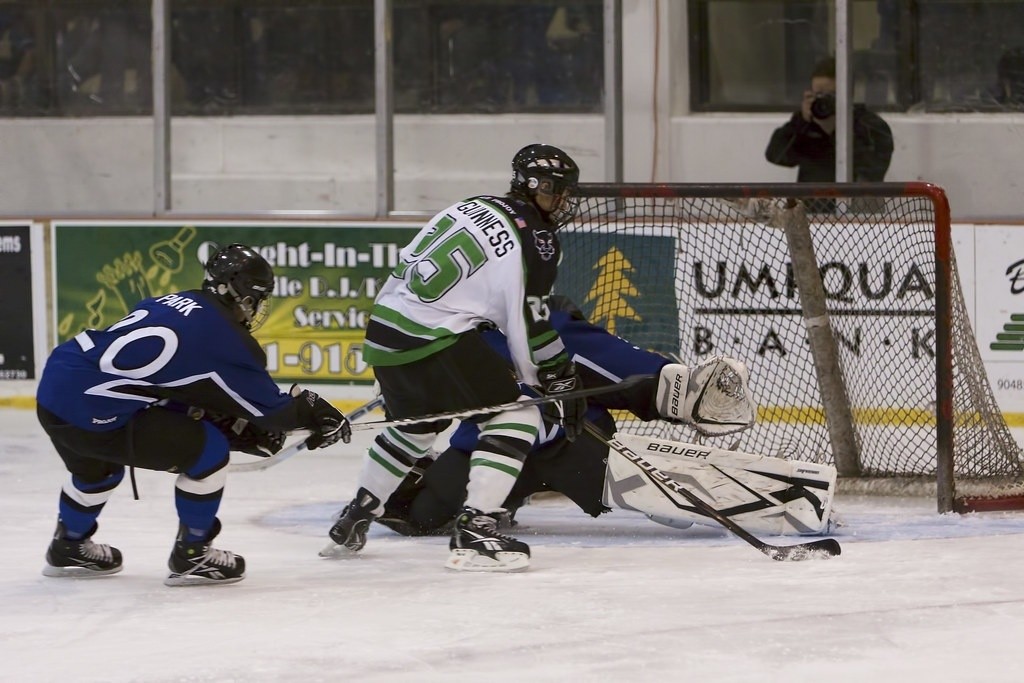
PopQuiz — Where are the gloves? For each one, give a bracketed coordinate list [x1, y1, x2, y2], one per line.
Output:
[226, 414, 286, 458]
[294, 389, 351, 451]
[543, 370, 589, 442]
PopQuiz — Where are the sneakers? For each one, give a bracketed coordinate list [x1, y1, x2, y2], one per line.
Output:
[42, 513, 123, 577]
[164, 515, 246, 585]
[444, 504, 531, 573]
[318, 486, 382, 557]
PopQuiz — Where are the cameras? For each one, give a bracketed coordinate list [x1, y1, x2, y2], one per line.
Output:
[810, 91, 837, 119]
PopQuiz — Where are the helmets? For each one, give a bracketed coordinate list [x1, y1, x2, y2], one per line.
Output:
[201, 244, 275, 312]
[512, 144, 580, 196]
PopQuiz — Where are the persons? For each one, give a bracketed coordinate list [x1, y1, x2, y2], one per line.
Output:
[398, 294, 761, 538]
[765, 55, 896, 217]
[0, 0, 605, 116]
[327, 144, 580, 558]
[35, 243, 353, 581]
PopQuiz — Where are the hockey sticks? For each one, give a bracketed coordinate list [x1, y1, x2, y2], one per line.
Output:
[521, 364, 842, 563]
[230, 394, 384, 472]
[348, 372, 658, 432]
